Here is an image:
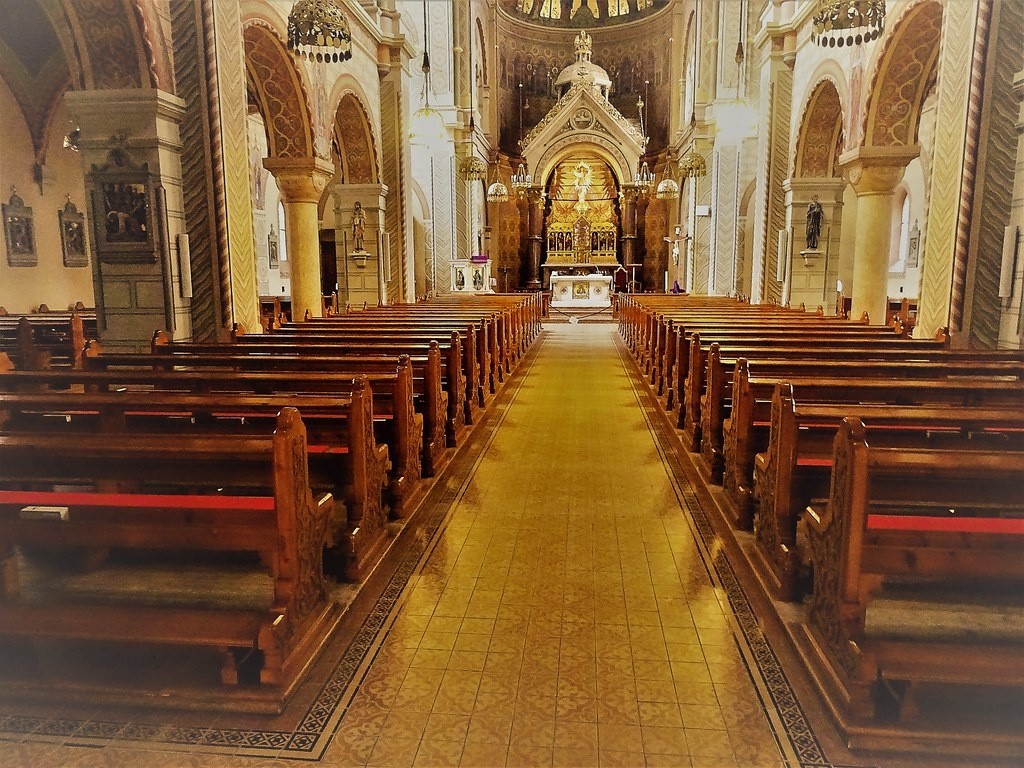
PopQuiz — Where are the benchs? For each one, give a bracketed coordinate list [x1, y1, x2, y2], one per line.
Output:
[618, 291, 1024, 768]
[0, 293, 543, 716]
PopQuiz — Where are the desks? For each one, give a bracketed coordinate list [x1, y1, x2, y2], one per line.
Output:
[549, 275, 613, 312]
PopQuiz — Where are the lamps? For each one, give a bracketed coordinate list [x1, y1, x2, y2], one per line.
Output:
[677, 0, 708, 179]
[458, 0, 487, 182]
[487, 0, 531, 204]
[287, 0, 354, 64]
[809, 0, 887, 47]
[408, 0, 449, 147]
[633, 0, 680, 201]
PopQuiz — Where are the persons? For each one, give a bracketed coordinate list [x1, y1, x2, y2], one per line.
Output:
[456, 270, 464, 287]
[674, 280, 679, 293]
[474, 270, 482, 290]
[351, 201, 366, 251]
[664, 237, 689, 266]
[806, 193, 824, 248]
